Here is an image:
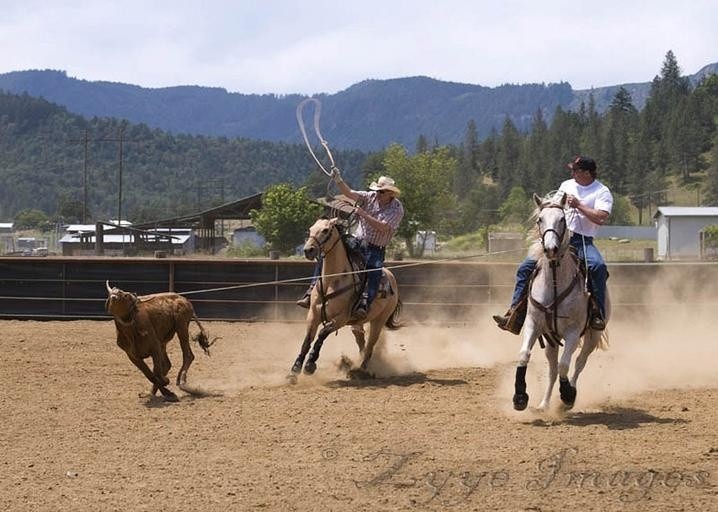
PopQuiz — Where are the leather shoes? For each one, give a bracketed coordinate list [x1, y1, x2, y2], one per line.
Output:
[589, 309, 604, 330]
[352, 304, 366, 319]
[297, 296, 310, 309]
[493, 314, 523, 335]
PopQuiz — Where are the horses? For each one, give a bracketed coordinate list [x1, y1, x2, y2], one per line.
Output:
[511, 191, 613, 417]
[284, 214, 406, 389]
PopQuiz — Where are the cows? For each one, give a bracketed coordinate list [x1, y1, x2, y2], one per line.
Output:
[103, 279, 221, 399]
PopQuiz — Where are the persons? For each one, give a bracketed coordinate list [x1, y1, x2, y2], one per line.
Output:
[296, 165, 403, 321]
[493, 155, 615, 333]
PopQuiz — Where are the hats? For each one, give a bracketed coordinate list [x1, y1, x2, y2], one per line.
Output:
[369, 176, 400, 198]
[568, 157, 594, 171]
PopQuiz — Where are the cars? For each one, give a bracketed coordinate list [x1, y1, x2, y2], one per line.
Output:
[34, 247, 48, 256]
[21, 248, 32, 256]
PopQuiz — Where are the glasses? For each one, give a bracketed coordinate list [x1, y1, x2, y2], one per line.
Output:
[375, 190, 391, 195]
